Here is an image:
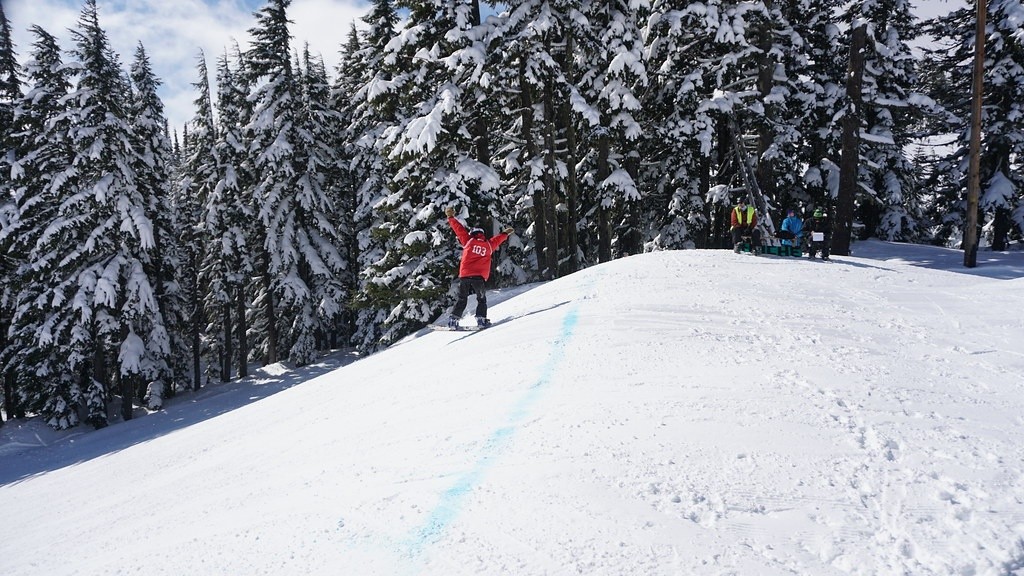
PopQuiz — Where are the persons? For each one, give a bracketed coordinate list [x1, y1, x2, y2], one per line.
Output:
[780, 207, 803, 258]
[730, 196, 761, 256]
[801, 208, 832, 262]
[444, 206, 514, 329]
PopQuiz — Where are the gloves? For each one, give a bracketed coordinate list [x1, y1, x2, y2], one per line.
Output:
[502, 228, 515, 236]
[445, 206, 456, 218]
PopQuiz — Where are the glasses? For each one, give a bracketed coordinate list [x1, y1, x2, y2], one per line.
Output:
[737, 201, 744, 204]
[814, 218, 820, 221]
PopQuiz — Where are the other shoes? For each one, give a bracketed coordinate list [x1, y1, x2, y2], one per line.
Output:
[477, 317, 493, 328]
[808, 256, 815, 261]
[822, 256, 830, 261]
[448, 319, 459, 330]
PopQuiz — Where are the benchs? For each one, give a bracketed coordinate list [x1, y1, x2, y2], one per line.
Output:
[741, 234, 801, 257]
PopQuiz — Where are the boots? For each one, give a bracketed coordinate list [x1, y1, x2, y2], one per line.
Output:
[753, 247, 762, 256]
[734, 244, 741, 255]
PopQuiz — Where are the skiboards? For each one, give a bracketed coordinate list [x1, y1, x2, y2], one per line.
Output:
[808, 257, 832, 264]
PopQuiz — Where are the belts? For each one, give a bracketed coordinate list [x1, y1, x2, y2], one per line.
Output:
[464, 276, 483, 279]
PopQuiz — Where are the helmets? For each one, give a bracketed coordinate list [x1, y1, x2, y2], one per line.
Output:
[468, 226, 485, 235]
[737, 196, 750, 205]
[812, 212, 823, 218]
[788, 209, 796, 216]
[815, 208, 822, 212]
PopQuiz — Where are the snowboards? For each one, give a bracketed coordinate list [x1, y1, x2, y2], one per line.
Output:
[426, 323, 491, 331]
